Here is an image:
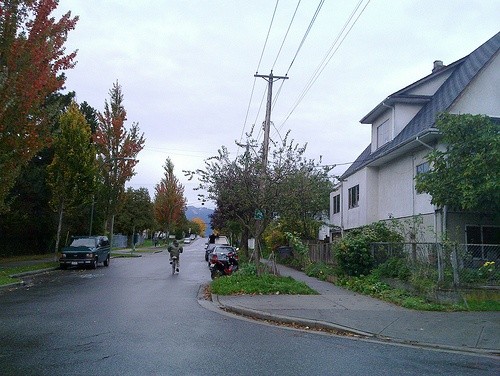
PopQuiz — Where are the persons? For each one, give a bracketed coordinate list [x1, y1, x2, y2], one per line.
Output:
[167, 240, 184, 273]
[152, 235, 159, 248]
[209, 232, 216, 244]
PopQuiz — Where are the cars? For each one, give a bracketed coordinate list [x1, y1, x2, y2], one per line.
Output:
[184, 238, 191, 244]
[209, 245, 238, 269]
[190, 235, 195, 240]
[205, 237, 230, 261]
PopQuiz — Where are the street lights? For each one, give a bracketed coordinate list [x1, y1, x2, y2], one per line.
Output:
[89, 157, 134, 235]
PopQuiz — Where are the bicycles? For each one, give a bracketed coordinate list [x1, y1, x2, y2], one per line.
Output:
[169, 250, 182, 275]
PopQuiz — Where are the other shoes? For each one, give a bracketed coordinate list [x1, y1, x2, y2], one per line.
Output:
[170, 262, 173, 264]
[176, 268, 179, 272]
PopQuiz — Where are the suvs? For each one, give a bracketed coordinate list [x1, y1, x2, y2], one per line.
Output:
[59, 235, 110, 268]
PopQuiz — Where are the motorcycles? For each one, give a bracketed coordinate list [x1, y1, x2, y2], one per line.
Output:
[208, 248, 239, 278]
